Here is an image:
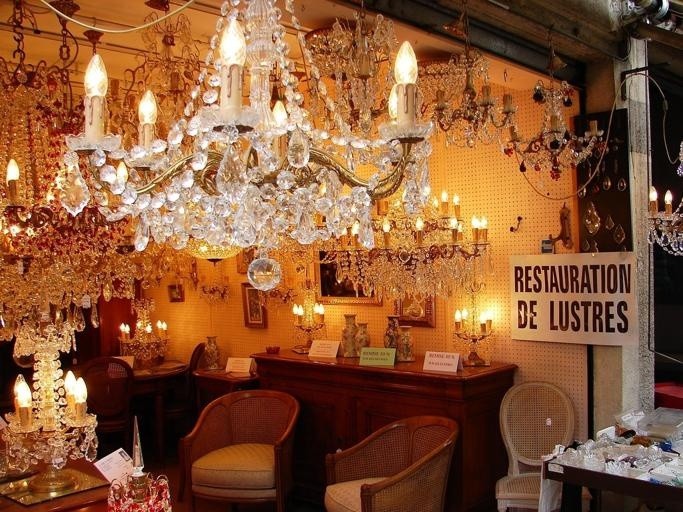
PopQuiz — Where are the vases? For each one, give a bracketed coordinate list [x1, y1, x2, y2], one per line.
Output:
[355, 322, 371, 357]
[340, 313, 359, 357]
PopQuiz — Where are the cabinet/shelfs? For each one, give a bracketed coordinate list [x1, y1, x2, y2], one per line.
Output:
[542, 408, 683, 511]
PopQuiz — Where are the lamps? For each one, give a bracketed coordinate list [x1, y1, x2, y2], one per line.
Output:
[453, 307, 495, 367]
[648, 185, 683, 261]
[133, 1, 205, 131]
[109, 416, 173, 512]
[10, 364, 99, 493]
[336, 0, 398, 135]
[504, 36, 606, 182]
[299, 23, 389, 134]
[291, 303, 326, 352]
[179, 239, 243, 270]
[0, 0, 119, 256]
[340, 191, 498, 304]
[57, 0, 423, 251]
[433, 3, 518, 149]
[3, 1, 131, 335]
[192, 278, 233, 306]
[115, 297, 169, 369]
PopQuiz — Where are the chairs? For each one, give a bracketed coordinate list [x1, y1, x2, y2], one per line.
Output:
[77, 355, 135, 459]
[496, 381, 592, 512]
[324, 415, 460, 512]
[180, 390, 299, 512]
[139, 338, 207, 460]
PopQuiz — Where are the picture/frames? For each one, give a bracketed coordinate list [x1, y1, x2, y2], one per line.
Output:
[392, 295, 436, 330]
[167, 284, 186, 303]
[241, 282, 268, 329]
[313, 240, 388, 306]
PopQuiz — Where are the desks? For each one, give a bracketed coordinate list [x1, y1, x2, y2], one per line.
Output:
[255, 345, 518, 511]
[193, 363, 260, 427]
